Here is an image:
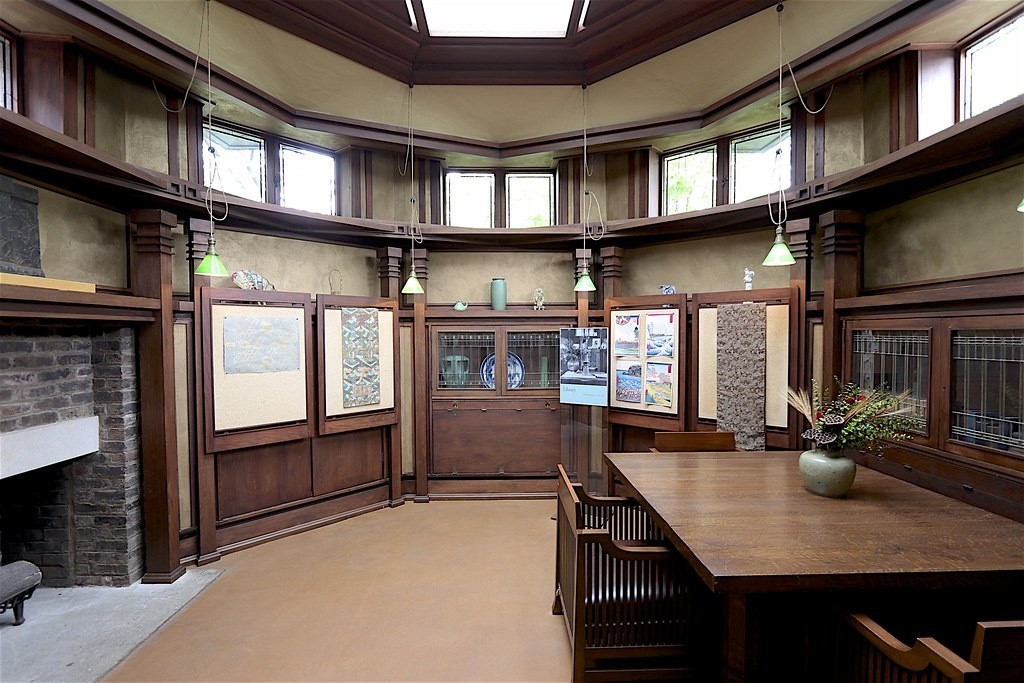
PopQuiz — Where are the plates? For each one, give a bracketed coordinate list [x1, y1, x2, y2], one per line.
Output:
[233, 270, 277, 292]
[480, 351, 525, 389]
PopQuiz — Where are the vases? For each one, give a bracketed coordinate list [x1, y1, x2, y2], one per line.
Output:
[800, 446, 856, 498]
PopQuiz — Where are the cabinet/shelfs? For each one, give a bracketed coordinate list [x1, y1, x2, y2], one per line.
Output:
[835, 312, 1024, 525]
[417, 312, 580, 474]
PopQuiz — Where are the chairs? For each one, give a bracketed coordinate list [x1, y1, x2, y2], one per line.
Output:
[653, 432, 734, 451]
[846, 608, 1024, 683]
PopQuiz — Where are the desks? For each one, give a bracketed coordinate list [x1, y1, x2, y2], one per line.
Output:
[601, 452, 1024, 683]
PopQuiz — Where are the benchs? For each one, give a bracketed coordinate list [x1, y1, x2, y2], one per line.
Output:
[555, 466, 717, 681]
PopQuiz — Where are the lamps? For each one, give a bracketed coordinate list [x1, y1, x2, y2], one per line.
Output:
[762, 1, 797, 268]
[402, 83, 425, 297]
[572, 78, 600, 293]
[192, 0, 232, 280]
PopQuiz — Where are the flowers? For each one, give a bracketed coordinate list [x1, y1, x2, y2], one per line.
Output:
[785, 377, 924, 451]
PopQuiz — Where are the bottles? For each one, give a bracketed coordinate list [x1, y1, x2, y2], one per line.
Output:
[491, 277, 507, 310]
